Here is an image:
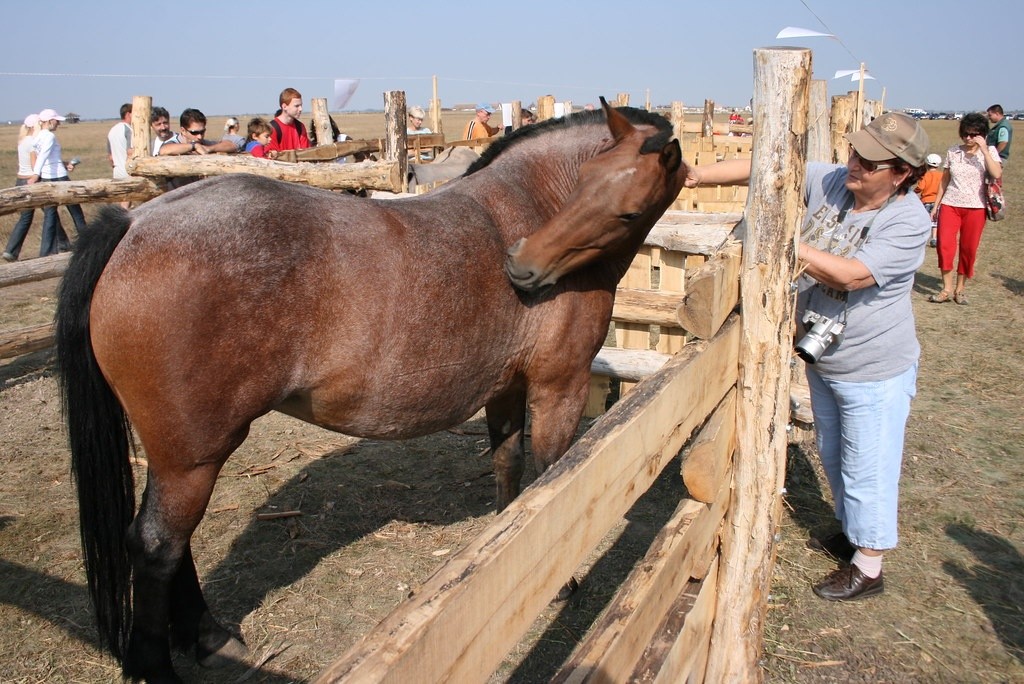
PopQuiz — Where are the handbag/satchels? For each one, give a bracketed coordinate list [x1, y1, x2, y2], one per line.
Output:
[984, 144, 1006, 221]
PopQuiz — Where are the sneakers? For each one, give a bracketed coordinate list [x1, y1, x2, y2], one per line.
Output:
[806, 531, 856, 561]
[814, 564, 885, 600]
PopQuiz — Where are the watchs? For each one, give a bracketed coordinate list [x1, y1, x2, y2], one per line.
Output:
[190, 142, 196, 151]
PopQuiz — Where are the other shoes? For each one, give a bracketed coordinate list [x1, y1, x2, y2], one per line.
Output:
[953, 290, 968, 305]
[928, 289, 953, 302]
[2, 254, 21, 262]
[930, 239, 937, 246]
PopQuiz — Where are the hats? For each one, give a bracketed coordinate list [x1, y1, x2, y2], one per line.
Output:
[841, 112, 930, 168]
[24, 113, 40, 127]
[927, 153, 942, 167]
[39, 108, 66, 121]
[475, 101, 496, 113]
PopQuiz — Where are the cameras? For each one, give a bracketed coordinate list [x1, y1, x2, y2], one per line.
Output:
[794, 309, 845, 364]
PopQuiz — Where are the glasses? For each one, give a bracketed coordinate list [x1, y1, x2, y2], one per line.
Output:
[849, 142, 903, 172]
[183, 126, 206, 135]
[961, 131, 981, 137]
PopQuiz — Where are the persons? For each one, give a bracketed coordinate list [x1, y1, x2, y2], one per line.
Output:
[984, 104, 1013, 171]
[729, 109, 744, 124]
[2, 108, 89, 263]
[406, 106, 433, 161]
[682, 112, 933, 602]
[913, 154, 944, 247]
[505, 109, 533, 136]
[463, 103, 504, 157]
[930, 113, 1003, 306]
[149, 87, 367, 197]
[106, 103, 135, 211]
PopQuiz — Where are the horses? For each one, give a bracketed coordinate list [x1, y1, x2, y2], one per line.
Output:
[56, 94, 689, 684]
